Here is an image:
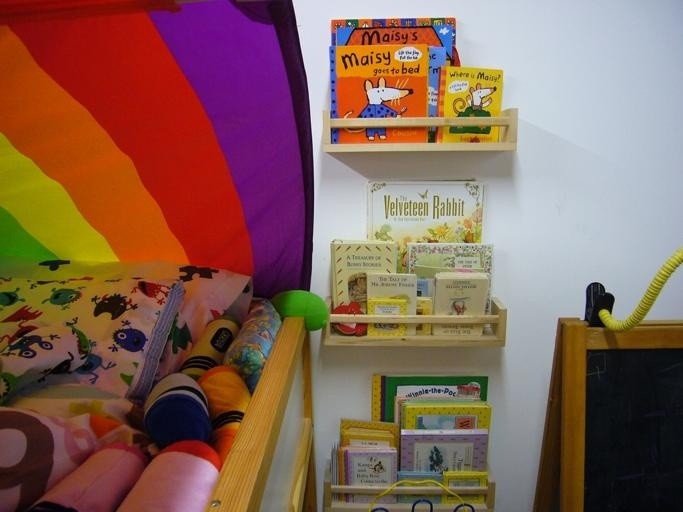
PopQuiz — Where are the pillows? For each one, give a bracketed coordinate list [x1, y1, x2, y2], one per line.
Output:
[0, 260, 256, 404]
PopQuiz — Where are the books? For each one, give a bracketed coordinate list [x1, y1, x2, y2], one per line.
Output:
[330, 374, 492, 504]
[329, 14, 503, 143]
[331, 180, 493, 337]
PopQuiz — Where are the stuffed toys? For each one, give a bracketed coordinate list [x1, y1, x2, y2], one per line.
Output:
[28, 280, 331, 511]
[27, 280, 330, 511]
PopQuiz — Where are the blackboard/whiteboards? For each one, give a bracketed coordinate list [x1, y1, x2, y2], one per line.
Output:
[558, 321, 683, 512]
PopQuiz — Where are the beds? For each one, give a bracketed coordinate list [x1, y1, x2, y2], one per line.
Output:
[0, 260, 316, 512]
